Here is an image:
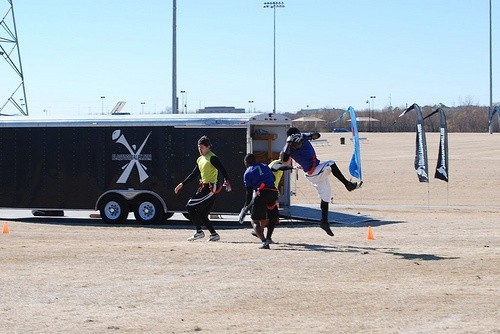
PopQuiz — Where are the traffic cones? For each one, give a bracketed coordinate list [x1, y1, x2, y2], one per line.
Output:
[367, 225, 375, 240]
[3, 222, 10, 233]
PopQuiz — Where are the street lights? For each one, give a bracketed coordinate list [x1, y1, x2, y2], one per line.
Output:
[370, 96, 376, 118]
[101, 96, 107, 116]
[141, 102, 146, 115]
[180, 91, 186, 114]
[262, 1, 286, 113]
[249, 101, 254, 112]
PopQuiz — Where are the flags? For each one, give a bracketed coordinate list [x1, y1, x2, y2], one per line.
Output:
[399, 103, 429, 182]
[423, 108, 448, 182]
[338, 106, 361, 179]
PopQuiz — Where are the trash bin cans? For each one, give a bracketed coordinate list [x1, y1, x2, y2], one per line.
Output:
[341, 137, 344, 144]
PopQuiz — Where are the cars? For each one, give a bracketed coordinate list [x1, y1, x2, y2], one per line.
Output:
[333, 128, 349, 132]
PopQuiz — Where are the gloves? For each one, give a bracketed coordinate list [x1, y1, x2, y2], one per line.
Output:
[286, 134, 307, 144]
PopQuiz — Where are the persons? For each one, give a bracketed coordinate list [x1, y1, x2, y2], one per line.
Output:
[239, 157, 301, 238]
[244, 153, 280, 249]
[280, 127, 363, 236]
[175, 136, 231, 241]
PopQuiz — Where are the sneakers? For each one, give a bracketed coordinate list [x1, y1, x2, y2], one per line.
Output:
[320, 223, 334, 236]
[208, 232, 220, 241]
[346, 180, 363, 192]
[187, 231, 205, 241]
[239, 208, 247, 224]
[259, 243, 269, 249]
[251, 229, 258, 237]
[267, 239, 274, 244]
